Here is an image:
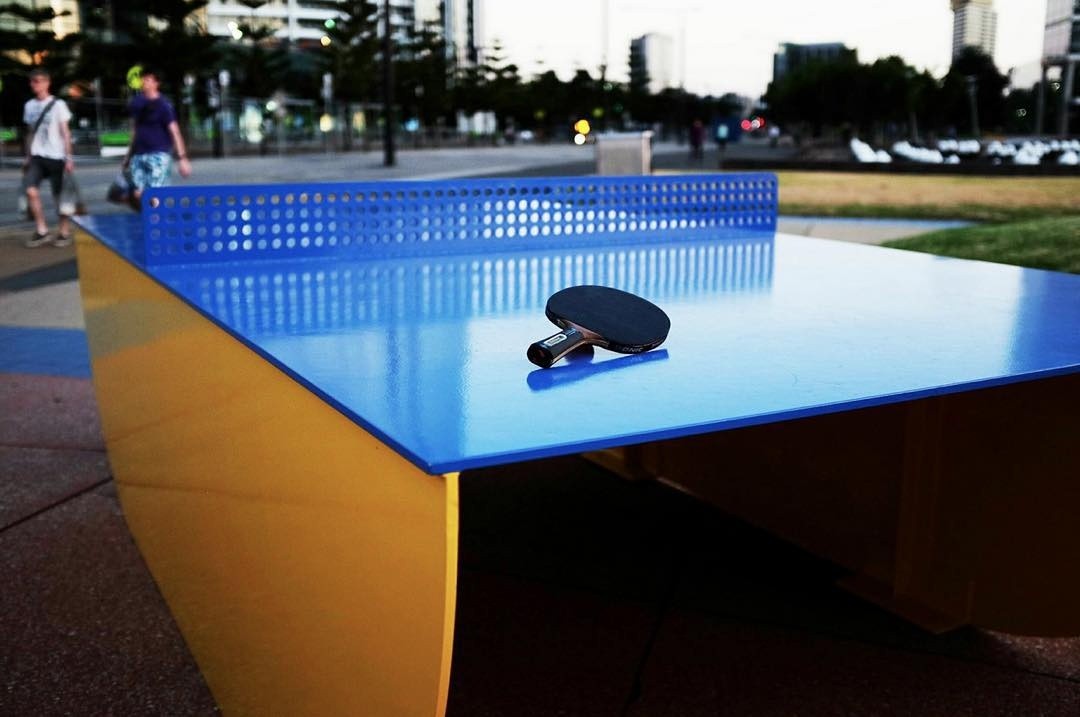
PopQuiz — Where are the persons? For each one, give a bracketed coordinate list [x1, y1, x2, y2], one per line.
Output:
[381, 103, 402, 168]
[20, 69, 74, 248]
[122, 64, 193, 213]
[350, 102, 368, 152]
[320, 107, 340, 155]
[688, 120, 709, 160]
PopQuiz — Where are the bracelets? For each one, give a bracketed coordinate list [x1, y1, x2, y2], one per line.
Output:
[64, 155, 72, 160]
[177, 153, 187, 160]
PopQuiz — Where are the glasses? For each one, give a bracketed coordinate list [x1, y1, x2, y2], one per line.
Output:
[30, 76, 46, 83]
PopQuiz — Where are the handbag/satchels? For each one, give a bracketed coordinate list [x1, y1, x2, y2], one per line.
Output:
[58, 170, 88, 217]
[105, 165, 133, 202]
[16, 170, 35, 221]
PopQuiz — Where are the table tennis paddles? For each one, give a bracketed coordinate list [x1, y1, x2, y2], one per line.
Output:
[525, 282, 672, 370]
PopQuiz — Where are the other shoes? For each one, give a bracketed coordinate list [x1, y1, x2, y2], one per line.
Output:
[55, 232, 73, 247]
[26, 230, 52, 247]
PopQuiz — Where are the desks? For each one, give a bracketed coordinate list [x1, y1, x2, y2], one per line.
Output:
[63, 172, 1080, 717]
